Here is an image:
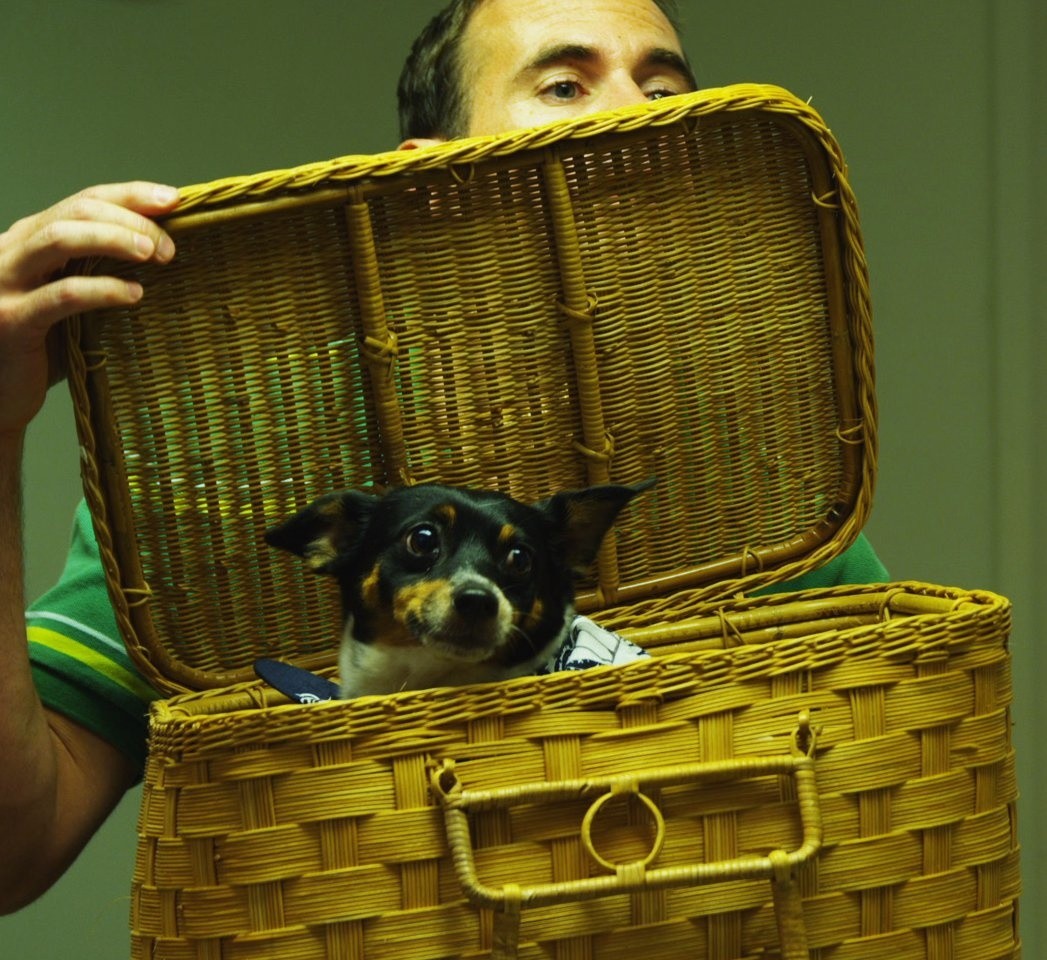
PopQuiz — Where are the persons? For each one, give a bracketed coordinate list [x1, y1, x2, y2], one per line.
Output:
[0, 0, 891, 916]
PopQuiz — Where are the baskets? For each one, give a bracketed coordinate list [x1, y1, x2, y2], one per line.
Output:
[131, 583, 1018, 960]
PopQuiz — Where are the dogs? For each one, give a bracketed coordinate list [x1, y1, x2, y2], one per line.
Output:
[260, 476, 659, 701]
